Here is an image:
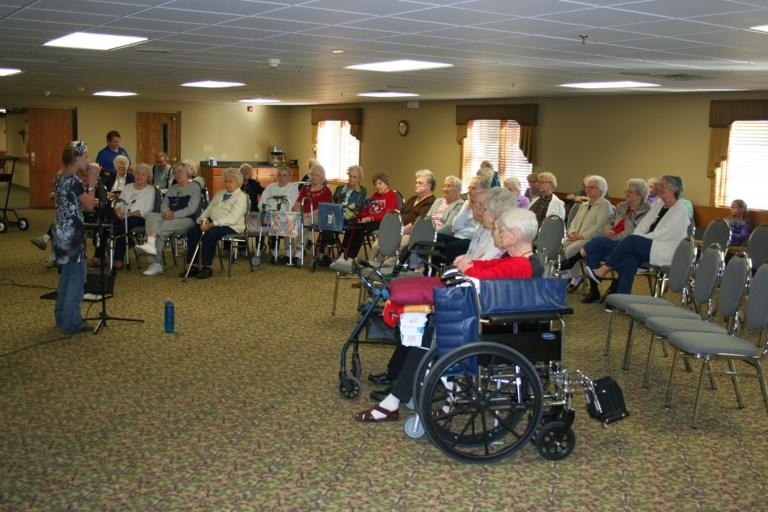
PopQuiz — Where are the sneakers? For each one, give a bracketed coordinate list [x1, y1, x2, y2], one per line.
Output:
[330, 255, 359, 273]
[30, 235, 47, 249]
[556, 260, 602, 302]
[143, 264, 163, 276]
[46, 255, 56, 268]
[135, 244, 157, 256]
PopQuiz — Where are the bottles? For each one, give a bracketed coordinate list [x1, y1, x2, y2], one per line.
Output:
[164, 297, 174, 333]
[210, 157, 214, 165]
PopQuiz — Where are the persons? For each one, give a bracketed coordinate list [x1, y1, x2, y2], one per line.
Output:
[29, 159, 104, 271]
[727, 199, 749, 246]
[47, 139, 103, 336]
[94, 132, 694, 304]
[353, 209, 544, 422]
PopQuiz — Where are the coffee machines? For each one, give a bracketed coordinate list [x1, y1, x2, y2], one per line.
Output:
[269, 146, 287, 165]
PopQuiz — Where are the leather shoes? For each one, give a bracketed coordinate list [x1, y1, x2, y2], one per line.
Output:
[368, 390, 391, 401]
[354, 403, 399, 422]
[272, 254, 301, 266]
[179, 266, 198, 277]
[198, 267, 213, 279]
[76, 324, 94, 332]
[368, 369, 399, 385]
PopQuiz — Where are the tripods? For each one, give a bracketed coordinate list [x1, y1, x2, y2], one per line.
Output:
[82, 176, 145, 335]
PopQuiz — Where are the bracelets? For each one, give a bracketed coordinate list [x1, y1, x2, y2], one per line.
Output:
[86, 186, 97, 193]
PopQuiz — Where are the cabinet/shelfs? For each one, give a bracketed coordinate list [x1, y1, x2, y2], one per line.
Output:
[201, 166, 299, 203]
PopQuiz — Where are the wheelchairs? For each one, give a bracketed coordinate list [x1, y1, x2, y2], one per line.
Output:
[413, 270, 575, 463]
[330, 242, 448, 397]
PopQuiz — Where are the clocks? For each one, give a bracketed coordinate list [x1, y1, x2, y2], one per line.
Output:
[398, 119, 408, 136]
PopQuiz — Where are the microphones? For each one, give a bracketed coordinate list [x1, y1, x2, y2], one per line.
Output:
[87, 163, 112, 176]
